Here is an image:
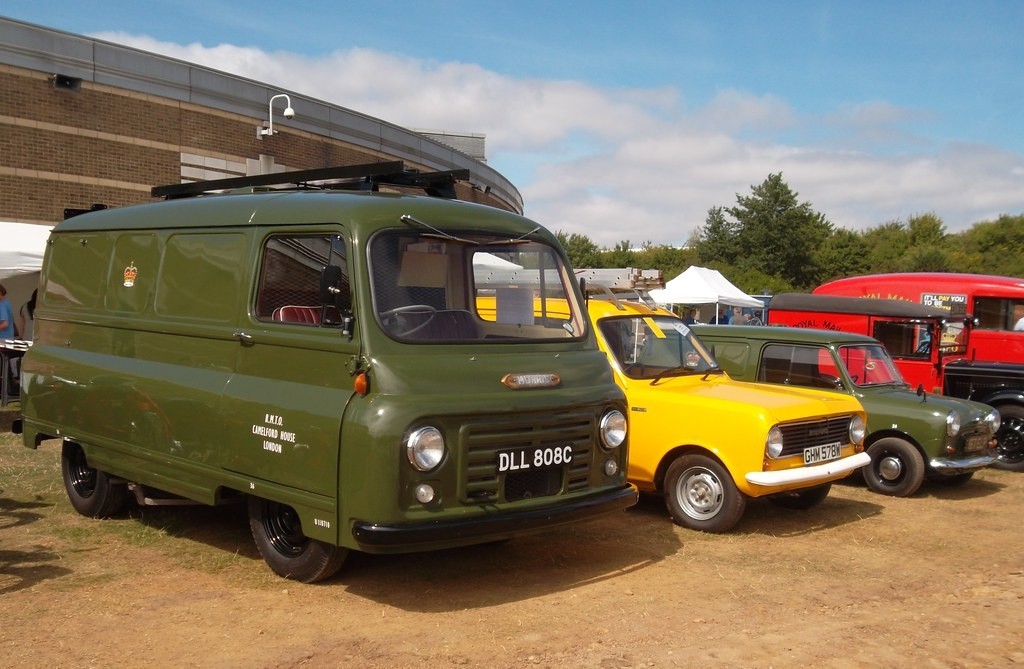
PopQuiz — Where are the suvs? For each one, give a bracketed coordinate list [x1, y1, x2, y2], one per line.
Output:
[812, 273, 1024, 366]
[767, 293, 1024, 471]
[475, 267, 871, 533]
[638, 325, 1003, 498]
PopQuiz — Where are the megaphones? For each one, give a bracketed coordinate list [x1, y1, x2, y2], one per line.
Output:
[52, 73, 82, 93]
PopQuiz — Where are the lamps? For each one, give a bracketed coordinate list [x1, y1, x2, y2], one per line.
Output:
[48, 72, 81, 93]
[472, 184, 491, 194]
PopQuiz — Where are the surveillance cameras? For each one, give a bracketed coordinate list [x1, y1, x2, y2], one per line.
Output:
[283, 108, 294, 119]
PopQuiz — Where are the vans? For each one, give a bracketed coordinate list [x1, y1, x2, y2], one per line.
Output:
[12, 160, 639, 582]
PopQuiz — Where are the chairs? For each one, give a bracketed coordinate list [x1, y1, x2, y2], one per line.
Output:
[396, 309, 478, 341]
[272, 305, 343, 325]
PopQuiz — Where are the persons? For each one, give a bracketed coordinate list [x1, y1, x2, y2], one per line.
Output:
[749, 311, 763, 326]
[0, 285, 20, 395]
[685, 309, 696, 325]
[729, 306, 747, 325]
[18, 288, 38, 343]
[710, 309, 729, 324]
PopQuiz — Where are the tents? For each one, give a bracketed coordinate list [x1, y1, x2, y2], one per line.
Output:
[639, 266, 764, 325]
[0, 221, 57, 285]
